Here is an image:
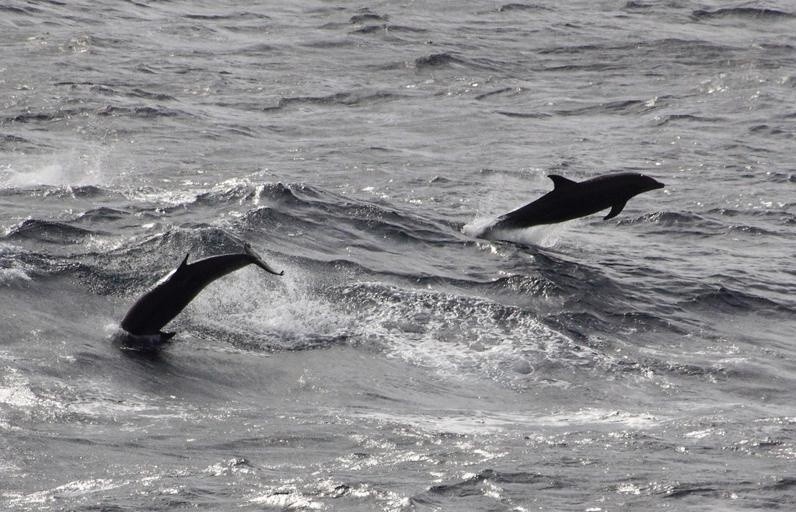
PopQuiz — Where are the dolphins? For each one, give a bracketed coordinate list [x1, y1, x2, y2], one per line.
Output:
[488, 171, 665, 231]
[119, 243, 285, 340]
